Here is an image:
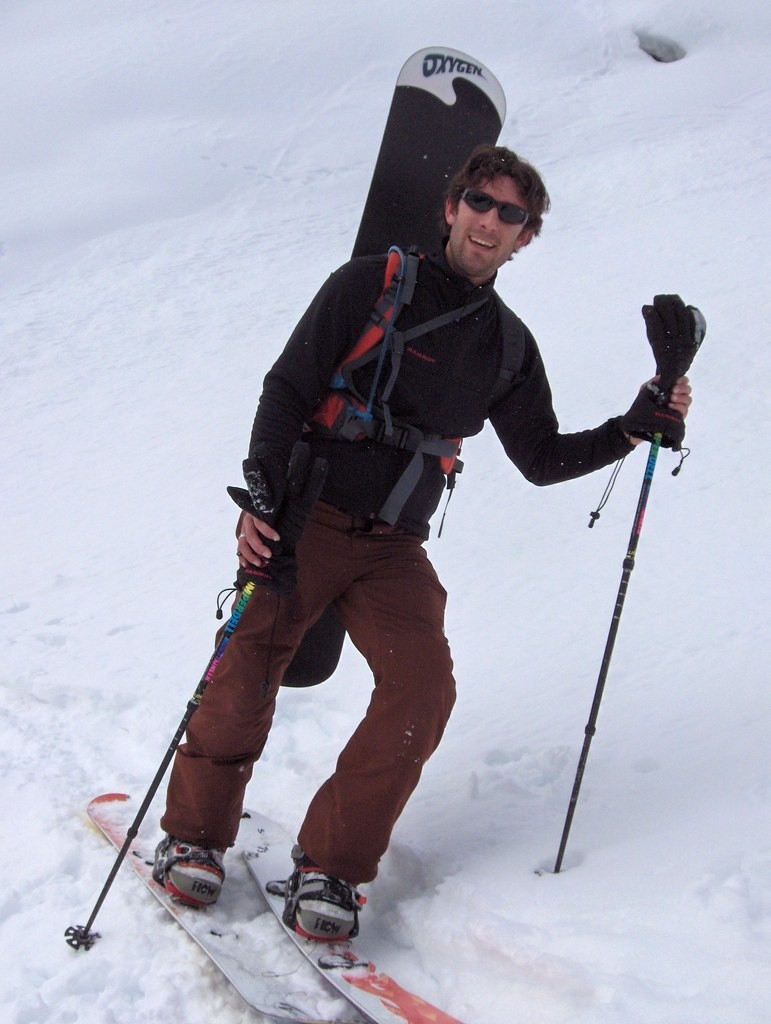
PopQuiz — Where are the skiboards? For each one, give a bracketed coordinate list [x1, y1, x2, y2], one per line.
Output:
[87, 793, 462, 1024]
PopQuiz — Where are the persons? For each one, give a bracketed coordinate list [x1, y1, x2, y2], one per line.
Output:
[155, 143, 694, 942]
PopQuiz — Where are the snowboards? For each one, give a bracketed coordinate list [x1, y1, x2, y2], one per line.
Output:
[271, 33, 508, 694]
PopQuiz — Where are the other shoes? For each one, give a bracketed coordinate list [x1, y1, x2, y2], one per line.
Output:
[281, 844, 367, 938]
[153, 832, 228, 906]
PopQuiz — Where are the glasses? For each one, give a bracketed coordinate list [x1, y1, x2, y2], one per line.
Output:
[456, 188, 540, 224]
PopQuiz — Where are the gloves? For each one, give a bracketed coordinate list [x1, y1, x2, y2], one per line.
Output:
[226, 442, 328, 591]
[620, 293, 707, 452]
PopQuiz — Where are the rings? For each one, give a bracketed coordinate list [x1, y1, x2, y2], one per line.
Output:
[238, 532, 246, 538]
